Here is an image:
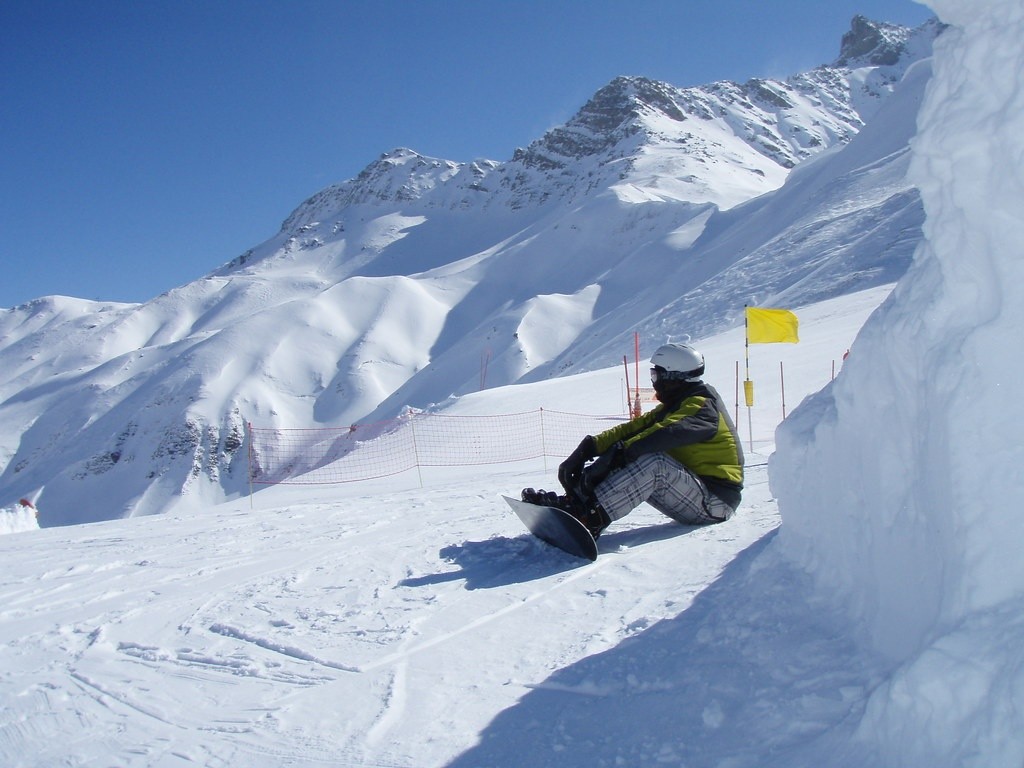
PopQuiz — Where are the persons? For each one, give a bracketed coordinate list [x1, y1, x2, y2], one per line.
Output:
[521, 343, 746, 543]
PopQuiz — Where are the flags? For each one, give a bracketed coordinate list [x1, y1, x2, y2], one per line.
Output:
[743, 306, 801, 345]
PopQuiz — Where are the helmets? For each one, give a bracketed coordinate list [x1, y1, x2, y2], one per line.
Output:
[650, 343, 705, 383]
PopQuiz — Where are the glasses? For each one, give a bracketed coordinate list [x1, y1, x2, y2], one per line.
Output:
[651, 368, 673, 383]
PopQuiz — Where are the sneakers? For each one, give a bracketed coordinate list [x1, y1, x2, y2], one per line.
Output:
[524, 491, 575, 514]
[555, 498, 599, 544]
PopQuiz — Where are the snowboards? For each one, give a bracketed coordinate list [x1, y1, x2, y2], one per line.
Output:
[501, 494, 599, 561]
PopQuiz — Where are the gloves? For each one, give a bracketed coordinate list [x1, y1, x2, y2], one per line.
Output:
[559, 436, 597, 487]
[579, 441, 626, 495]
